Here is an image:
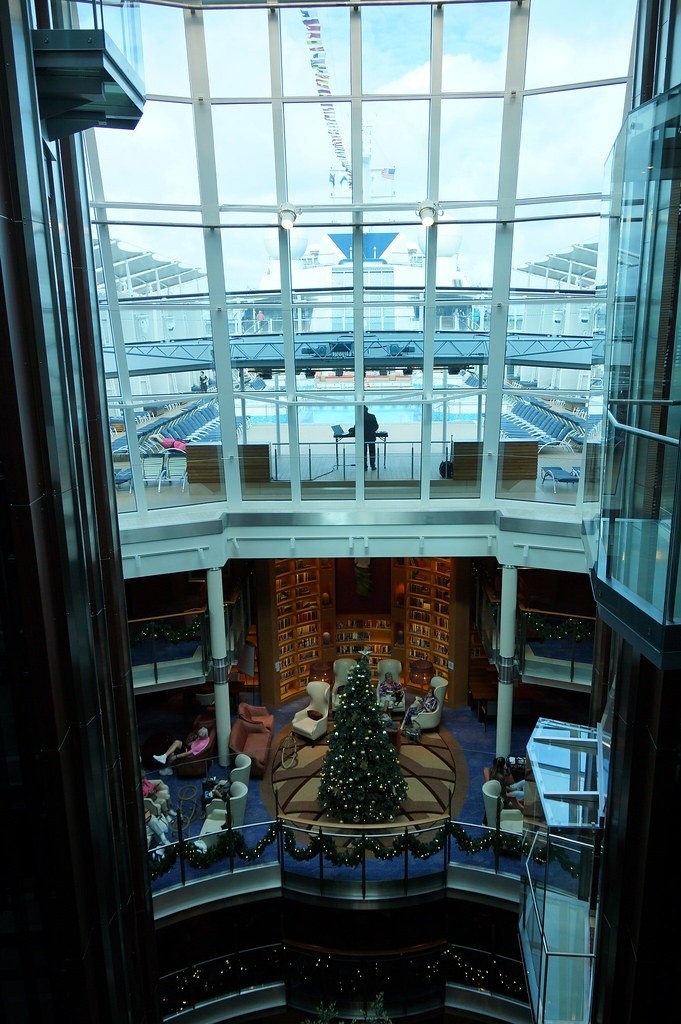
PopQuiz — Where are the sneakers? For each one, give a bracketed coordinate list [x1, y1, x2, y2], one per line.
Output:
[153, 755, 167, 764]
[159, 768, 173, 776]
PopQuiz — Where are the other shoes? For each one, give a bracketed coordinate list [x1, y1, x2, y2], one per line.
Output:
[166, 816, 175, 824]
[200, 811, 207, 820]
[161, 838, 170, 845]
[169, 809, 177, 816]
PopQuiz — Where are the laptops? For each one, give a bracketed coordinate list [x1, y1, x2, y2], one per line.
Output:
[332, 425, 349, 435]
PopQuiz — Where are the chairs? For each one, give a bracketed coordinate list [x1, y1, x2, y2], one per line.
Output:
[175, 725, 216, 775]
[192, 706, 215, 731]
[376, 659, 405, 718]
[482, 767, 492, 781]
[481, 779, 523, 835]
[198, 781, 248, 842]
[205, 754, 251, 818]
[332, 657, 357, 711]
[409, 676, 448, 731]
[109, 395, 254, 494]
[289, 681, 330, 747]
[464, 374, 588, 494]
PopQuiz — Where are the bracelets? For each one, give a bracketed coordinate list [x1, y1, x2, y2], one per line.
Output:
[176, 755, 177, 758]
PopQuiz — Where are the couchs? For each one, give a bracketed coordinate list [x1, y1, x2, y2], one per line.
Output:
[241, 705, 275, 736]
[231, 720, 272, 778]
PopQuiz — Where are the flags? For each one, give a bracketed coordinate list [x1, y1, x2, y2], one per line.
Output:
[382, 167, 395, 180]
[300, 9, 352, 190]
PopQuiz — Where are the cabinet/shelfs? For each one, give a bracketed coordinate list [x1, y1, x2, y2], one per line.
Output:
[255, 558, 325, 711]
[404, 557, 469, 709]
[334, 613, 392, 668]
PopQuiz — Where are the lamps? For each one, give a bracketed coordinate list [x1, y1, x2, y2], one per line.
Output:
[278, 202, 303, 229]
[415, 200, 444, 227]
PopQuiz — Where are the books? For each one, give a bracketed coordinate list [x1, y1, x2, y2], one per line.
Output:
[276, 560, 319, 695]
[409, 571, 450, 670]
[335, 618, 391, 676]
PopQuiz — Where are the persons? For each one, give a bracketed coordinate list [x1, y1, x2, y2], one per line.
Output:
[489, 757, 524, 799]
[453, 308, 480, 322]
[364, 405, 378, 471]
[140, 770, 177, 823]
[200, 777, 230, 820]
[149, 434, 186, 452]
[199, 370, 208, 391]
[145, 815, 170, 844]
[379, 672, 406, 719]
[153, 727, 209, 776]
[256, 311, 265, 330]
[399, 686, 437, 731]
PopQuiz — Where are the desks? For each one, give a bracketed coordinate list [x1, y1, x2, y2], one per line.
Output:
[334, 431, 390, 469]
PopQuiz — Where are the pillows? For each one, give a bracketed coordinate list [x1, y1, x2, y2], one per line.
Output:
[338, 684, 346, 695]
[307, 709, 323, 721]
